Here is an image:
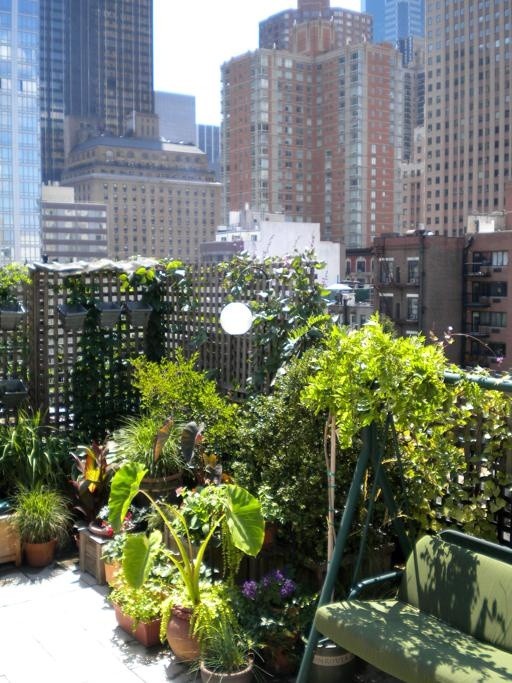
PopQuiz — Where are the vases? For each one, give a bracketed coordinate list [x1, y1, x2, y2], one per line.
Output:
[258, 639, 299, 675]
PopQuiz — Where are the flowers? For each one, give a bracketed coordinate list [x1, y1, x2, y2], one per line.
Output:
[239, 570, 300, 661]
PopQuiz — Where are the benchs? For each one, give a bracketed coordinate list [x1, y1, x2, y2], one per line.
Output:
[314, 529, 512, 683]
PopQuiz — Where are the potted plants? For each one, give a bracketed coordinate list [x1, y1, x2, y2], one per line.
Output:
[298, 591, 357, 683]
[109, 461, 266, 665]
[190, 592, 270, 682]
[0, 406, 223, 648]
